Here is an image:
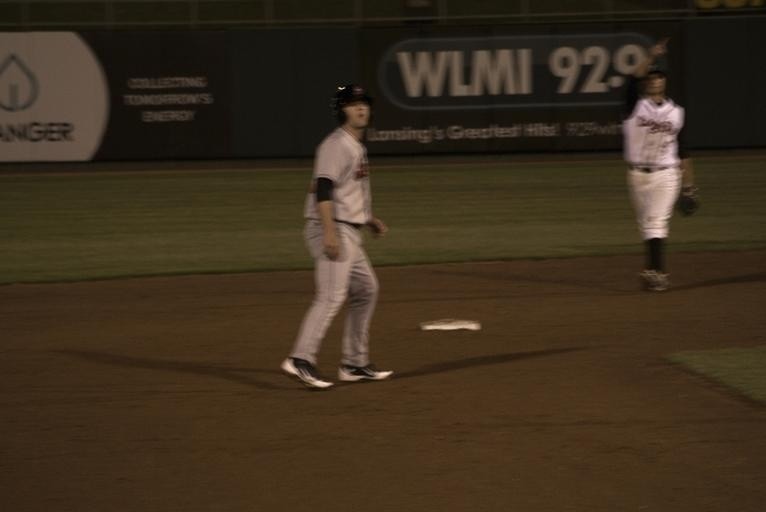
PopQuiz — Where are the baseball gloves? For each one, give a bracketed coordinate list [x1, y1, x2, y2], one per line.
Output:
[675, 187, 700, 216]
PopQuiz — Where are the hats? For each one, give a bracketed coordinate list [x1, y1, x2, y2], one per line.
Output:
[647, 63, 669, 78]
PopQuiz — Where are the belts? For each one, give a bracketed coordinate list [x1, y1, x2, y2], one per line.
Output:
[334, 218, 364, 229]
[628, 164, 670, 173]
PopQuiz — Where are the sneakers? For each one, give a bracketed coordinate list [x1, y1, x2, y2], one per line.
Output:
[679, 195, 698, 214]
[337, 364, 394, 381]
[280, 356, 335, 388]
[639, 269, 672, 291]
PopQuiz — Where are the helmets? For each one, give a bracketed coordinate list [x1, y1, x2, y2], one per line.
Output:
[333, 82, 373, 111]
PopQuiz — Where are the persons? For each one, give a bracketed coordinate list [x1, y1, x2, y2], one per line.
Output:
[279, 82, 393, 390]
[620, 42, 695, 292]
